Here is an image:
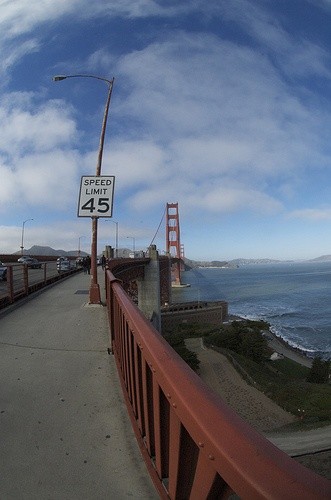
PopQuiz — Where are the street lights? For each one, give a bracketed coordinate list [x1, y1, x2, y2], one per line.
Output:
[77, 235, 82, 257]
[105, 218, 120, 258]
[20, 217, 35, 256]
[53, 72, 116, 304]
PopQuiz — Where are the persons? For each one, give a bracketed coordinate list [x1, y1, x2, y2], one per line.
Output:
[82, 255, 91, 275]
[101, 255, 106, 271]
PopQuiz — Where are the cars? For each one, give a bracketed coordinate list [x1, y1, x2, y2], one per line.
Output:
[76, 257, 84, 265]
[57, 256, 69, 262]
[23, 258, 42, 268]
[17, 255, 30, 263]
[56, 261, 71, 273]
[0, 260, 8, 281]
